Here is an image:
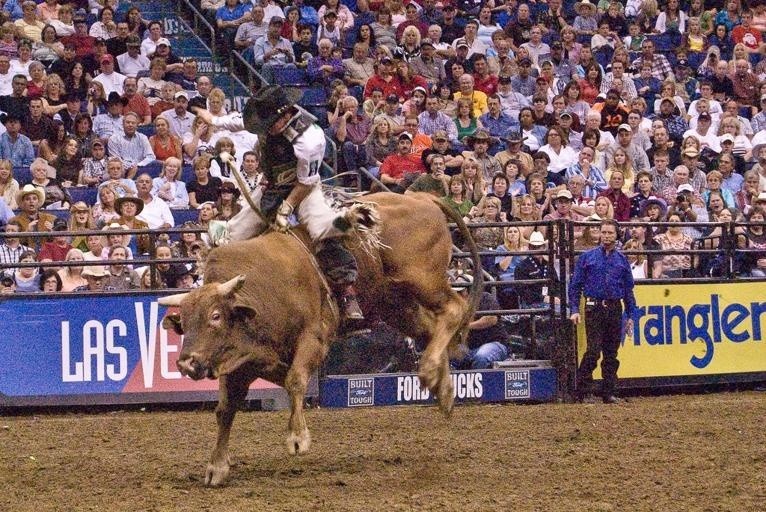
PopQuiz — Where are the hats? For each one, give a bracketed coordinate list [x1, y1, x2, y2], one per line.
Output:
[89, 138, 103, 150]
[69, 200, 92, 215]
[113, 192, 144, 216]
[420, 39, 436, 50]
[618, 122, 632, 134]
[455, 38, 469, 50]
[15, 184, 45, 211]
[52, 218, 68, 231]
[573, 0, 597, 15]
[398, 130, 529, 150]
[559, 108, 574, 123]
[268, 14, 283, 28]
[213, 181, 241, 204]
[379, 55, 393, 65]
[100, 222, 131, 248]
[175, 90, 188, 99]
[682, 148, 700, 157]
[242, 83, 305, 137]
[525, 184, 694, 251]
[324, 9, 337, 18]
[99, 32, 173, 65]
[100, 90, 130, 107]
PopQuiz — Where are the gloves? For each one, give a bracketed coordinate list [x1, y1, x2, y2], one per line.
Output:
[274, 199, 294, 235]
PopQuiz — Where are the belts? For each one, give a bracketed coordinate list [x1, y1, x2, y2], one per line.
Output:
[586, 297, 622, 307]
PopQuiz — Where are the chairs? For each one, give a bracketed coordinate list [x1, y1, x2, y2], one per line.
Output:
[0, 1, 329, 272]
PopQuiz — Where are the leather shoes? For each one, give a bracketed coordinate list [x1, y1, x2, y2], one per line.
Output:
[578, 395, 596, 403]
[602, 395, 620, 403]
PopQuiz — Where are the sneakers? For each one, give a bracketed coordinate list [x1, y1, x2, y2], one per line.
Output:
[333, 285, 365, 322]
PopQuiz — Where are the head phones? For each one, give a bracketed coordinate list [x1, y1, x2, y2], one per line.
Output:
[599, 219, 624, 240]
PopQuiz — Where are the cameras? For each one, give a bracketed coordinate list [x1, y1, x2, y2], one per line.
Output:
[276, 47, 287, 53]
[88, 88, 96, 96]
[675, 191, 687, 203]
[559, 109, 567, 114]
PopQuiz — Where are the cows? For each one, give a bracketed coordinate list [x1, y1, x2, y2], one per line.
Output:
[162, 189, 482, 488]
